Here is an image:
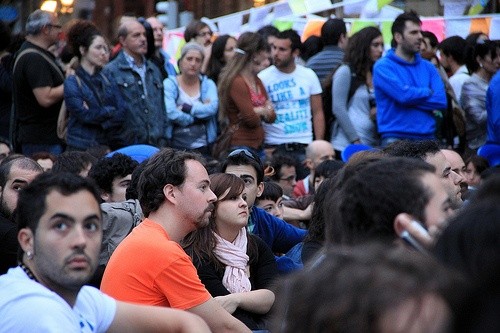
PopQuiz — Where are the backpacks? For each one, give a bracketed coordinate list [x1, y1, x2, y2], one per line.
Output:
[57, 71, 110, 139]
[323, 63, 366, 141]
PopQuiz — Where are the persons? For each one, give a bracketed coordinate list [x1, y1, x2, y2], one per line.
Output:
[1, 6, 500, 333]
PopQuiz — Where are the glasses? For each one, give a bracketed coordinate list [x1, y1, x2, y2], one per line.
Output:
[281, 176, 294, 183]
[225, 148, 262, 167]
[198, 31, 213, 36]
[47, 23, 62, 30]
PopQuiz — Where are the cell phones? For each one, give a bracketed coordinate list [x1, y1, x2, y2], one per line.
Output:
[400, 220, 430, 255]
